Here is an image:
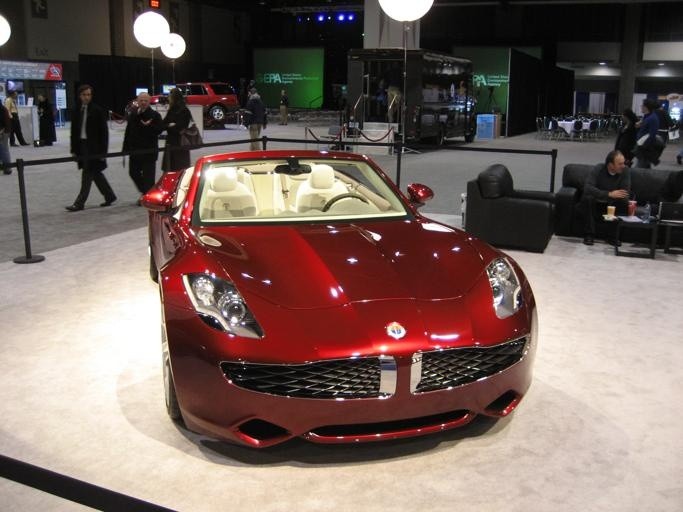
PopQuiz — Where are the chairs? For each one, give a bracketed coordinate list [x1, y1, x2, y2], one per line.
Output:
[203, 167, 257, 218]
[536, 114, 627, 141]
[465, 163, 555, 254]
[296, 165, 349, 211]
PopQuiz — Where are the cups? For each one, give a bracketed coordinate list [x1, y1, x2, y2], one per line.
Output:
[627, 200, 638, 217]
[607, 206, 616, 217]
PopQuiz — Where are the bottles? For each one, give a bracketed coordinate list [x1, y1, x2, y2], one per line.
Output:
[644, 201, 651, 218]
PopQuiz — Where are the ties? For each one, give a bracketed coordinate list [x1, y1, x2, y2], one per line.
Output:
[80, 106, 85, 126]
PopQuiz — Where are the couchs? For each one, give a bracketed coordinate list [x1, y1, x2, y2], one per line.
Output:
[556, 164, 683, 247]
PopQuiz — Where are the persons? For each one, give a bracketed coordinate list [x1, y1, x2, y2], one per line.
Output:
[0, 79, 290, 211]
[387, 72, 411, 123]
[571, 97, 683, 247]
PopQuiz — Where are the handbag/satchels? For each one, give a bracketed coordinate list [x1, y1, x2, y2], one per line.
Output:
[179, 107, 202, 150]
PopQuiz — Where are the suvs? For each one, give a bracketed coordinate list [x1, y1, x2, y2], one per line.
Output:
[122, 80, 242, 123]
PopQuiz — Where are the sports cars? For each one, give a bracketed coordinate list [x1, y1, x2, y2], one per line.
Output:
[137, 145, 541, 452]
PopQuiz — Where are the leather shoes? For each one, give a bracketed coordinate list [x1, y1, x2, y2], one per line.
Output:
[66, 205, 83, 211]
[11, 144, 18, 146]
[583, 236, 593, 244]
[100, 196, 116, 207]
[21, 143, 29, 145]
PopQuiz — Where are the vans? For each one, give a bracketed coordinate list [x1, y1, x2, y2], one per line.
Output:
[343, 45, 482, 151]
